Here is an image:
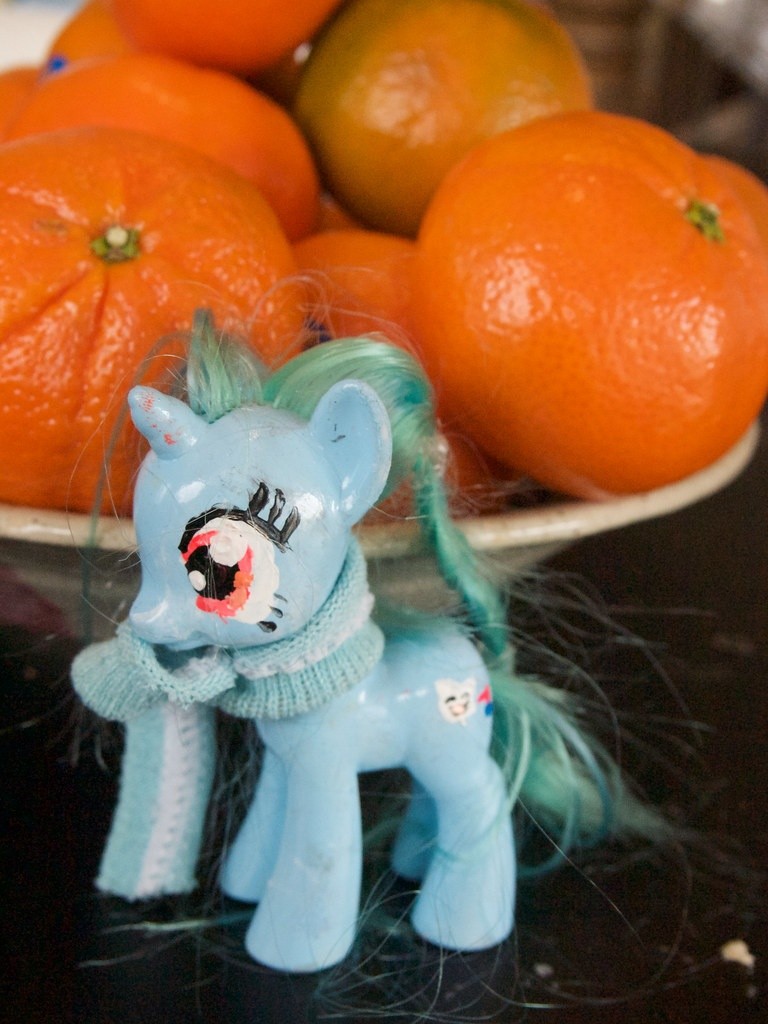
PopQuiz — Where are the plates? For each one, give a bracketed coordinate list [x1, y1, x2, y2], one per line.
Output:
[0, 62, 761, 642]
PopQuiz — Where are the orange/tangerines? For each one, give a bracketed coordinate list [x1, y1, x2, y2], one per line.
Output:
[92, 0, 334, 82]
[10, 55, 318, 242]
[1, 130, 308, 514]
[411, 112, 767, 499]
[293, 233, 485, 526]
[296, 0, 592, 241]
